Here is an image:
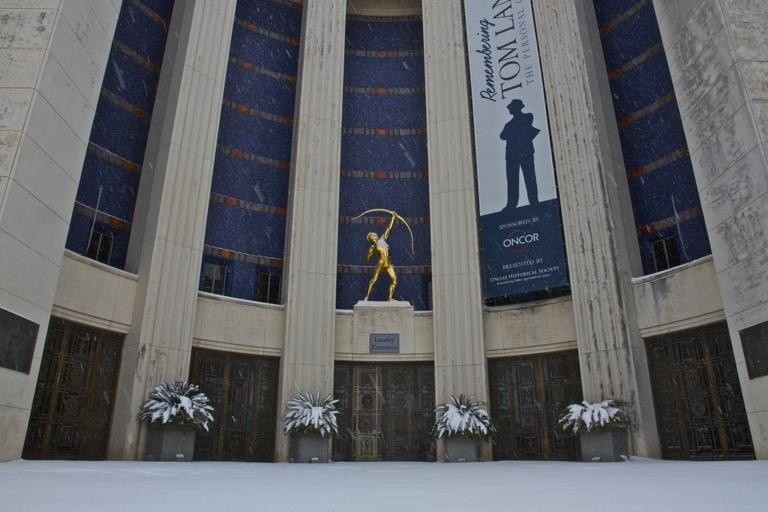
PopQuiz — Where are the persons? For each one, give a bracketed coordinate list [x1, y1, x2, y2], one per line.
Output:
[364, 211, 398, 301]
[500, 98, 541, 210]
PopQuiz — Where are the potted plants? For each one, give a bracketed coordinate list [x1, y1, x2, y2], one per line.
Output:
[555, 396, 636, 461]
[137, 379, 215, 463]
[428, 391, 498, 463]
[282, 389, 341, 462]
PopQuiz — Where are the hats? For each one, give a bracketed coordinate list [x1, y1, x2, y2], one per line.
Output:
[507, 99, 526, 109]
